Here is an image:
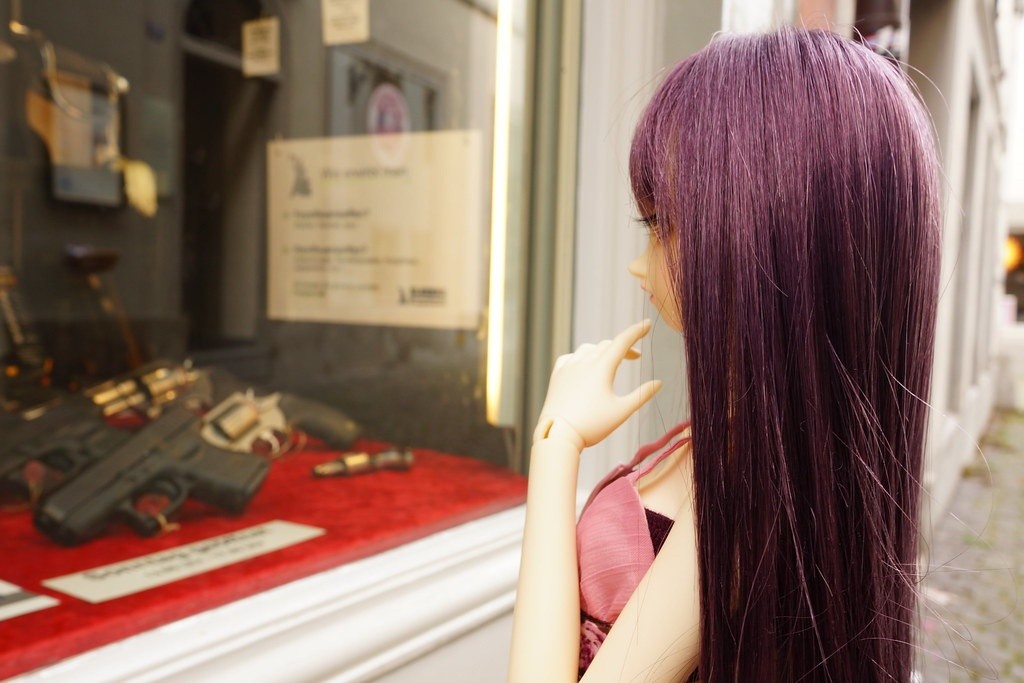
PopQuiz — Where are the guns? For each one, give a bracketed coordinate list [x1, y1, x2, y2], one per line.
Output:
[29, 403, 274, 548]
[0, 354, 361, 498]
